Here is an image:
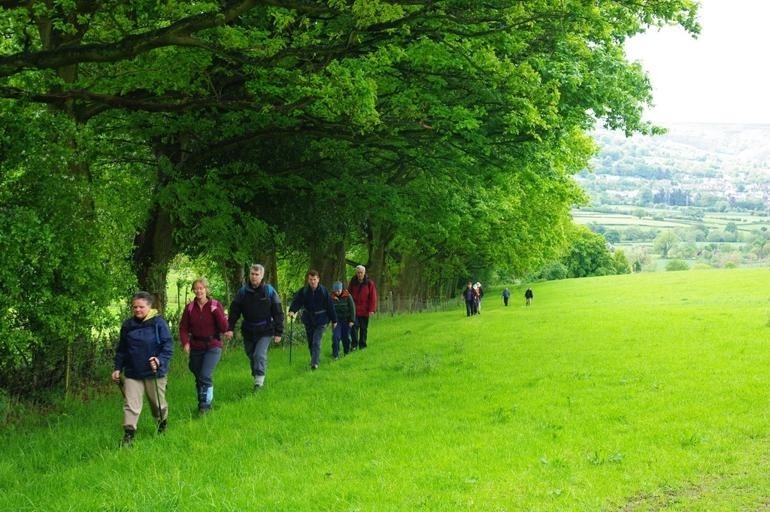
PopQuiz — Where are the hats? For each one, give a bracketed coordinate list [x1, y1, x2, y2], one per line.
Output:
[333, 284, 342, 289]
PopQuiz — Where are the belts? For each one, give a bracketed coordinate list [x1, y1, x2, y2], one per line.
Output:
[192, 336, 217, 342]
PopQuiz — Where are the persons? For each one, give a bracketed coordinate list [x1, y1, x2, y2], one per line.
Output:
[502, 287, 510, 305]
[330, 281, 357, 359]
[111, 292, 173, 446]
[348, 265, 377, 351]
[228, 264, 284, 391]
[461, 281, 483, 316]
[179, 280, 234, 411]
[525, 288, 532, 305]
[288, 270, 339, 369]
